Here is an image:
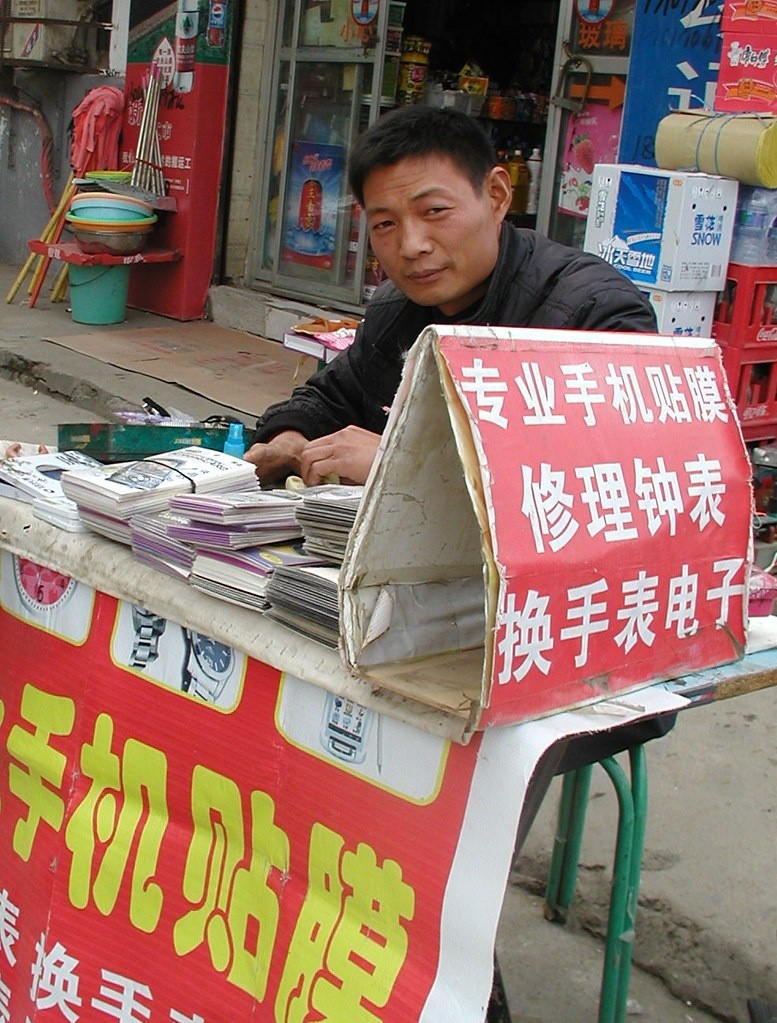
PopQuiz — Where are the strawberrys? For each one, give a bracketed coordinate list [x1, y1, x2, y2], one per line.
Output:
[575, 138, 595, 174]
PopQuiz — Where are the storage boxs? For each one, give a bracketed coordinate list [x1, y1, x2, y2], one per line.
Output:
[709, 261, 777, 443]
[428, 90, 516, 122]
[57, 423, 256, 463]
[581, 163, 740, 293]
[637, 285, 717, 338]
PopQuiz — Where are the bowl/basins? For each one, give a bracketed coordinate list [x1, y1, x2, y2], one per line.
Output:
[66, 192, 157, 256]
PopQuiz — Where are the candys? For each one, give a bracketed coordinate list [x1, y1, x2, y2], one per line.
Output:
[401, 39, 431, 61]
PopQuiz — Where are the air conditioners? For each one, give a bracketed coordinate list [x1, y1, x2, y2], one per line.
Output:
[2, 0, 97, 65]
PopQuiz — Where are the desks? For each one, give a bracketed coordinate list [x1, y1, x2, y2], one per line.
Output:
[0, 491, 776, 1023]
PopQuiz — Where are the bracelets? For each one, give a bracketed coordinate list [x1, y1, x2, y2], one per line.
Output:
[127, 604, 166, 670]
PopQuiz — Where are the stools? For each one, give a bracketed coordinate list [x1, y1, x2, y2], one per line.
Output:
[541, 744, 648, 1023]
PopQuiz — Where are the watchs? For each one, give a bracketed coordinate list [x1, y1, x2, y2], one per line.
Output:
[185, 629, 235, 704]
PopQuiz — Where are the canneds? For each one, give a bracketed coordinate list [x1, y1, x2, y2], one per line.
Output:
[298, 178, 322, 231]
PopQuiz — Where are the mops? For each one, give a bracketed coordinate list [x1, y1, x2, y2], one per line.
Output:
[5, 85, 126, 308]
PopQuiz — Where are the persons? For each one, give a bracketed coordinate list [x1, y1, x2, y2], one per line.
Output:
[240, 102, 677, 1023]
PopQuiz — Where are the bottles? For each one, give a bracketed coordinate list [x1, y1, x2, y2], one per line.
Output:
[207, 0, 227, 47]
[527, 148, 542, 214]
[506, 150, 531, 217]
[730, 187, 777, 266]
[223, 423, 245, 460]
[173, 0, 199, 93]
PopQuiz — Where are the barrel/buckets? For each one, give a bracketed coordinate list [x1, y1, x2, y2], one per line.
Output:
[67, 263, 130, 325]
